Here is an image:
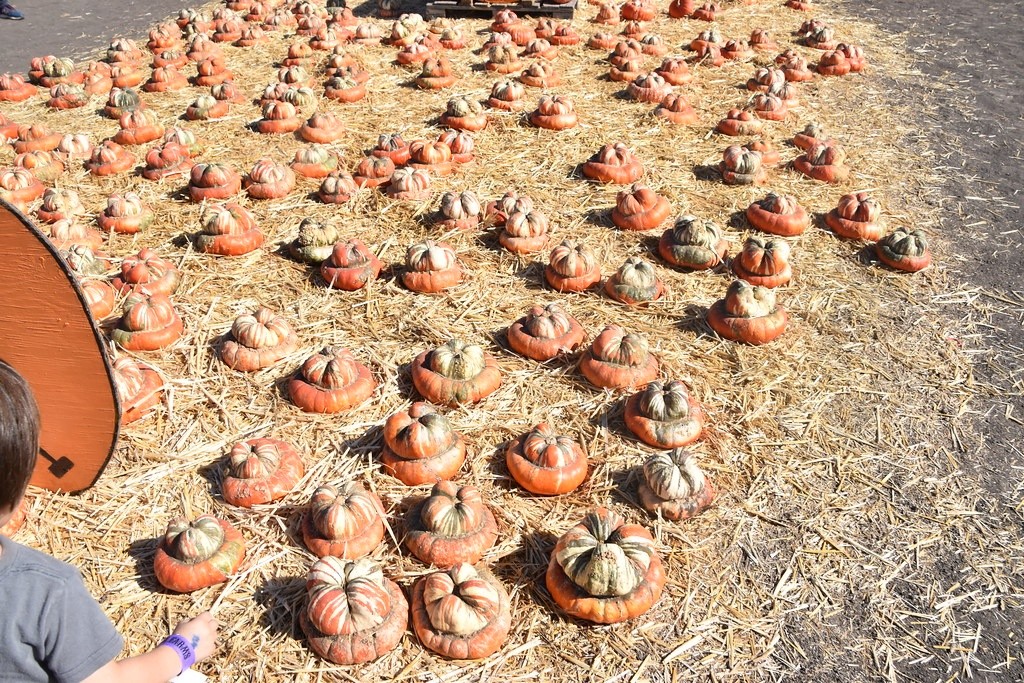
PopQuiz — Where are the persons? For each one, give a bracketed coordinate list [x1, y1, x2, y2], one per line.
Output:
[0, 357, 220, 683]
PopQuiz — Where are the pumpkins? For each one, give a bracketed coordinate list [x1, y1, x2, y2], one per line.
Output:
[0, 0, 930, 666]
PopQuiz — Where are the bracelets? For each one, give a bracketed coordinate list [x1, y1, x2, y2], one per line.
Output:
[160, 634, 195, 675]
